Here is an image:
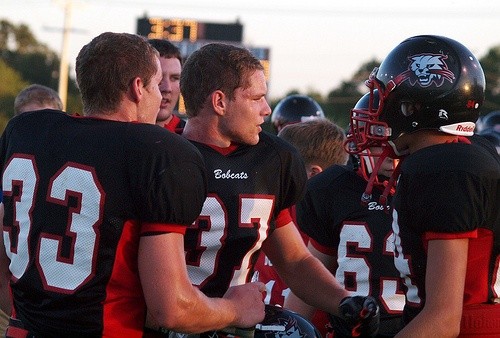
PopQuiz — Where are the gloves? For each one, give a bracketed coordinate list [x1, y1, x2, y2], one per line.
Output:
[339, 294, 379, 337]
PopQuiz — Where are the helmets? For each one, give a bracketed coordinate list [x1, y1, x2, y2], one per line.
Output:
[352, 88, 379, 141]
[369, 34, 487, 137]
[479, 109, 500, 155]
[254, 303, 322, 338]
[270, 94, 326, 137]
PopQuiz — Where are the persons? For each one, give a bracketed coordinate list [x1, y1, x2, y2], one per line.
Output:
[366, 36, 500, 338]
[143, 43, 380, 338]
[13, 85, 62, 115]
[0, 32, 266, 338]
[150, 39, 401, 338]
